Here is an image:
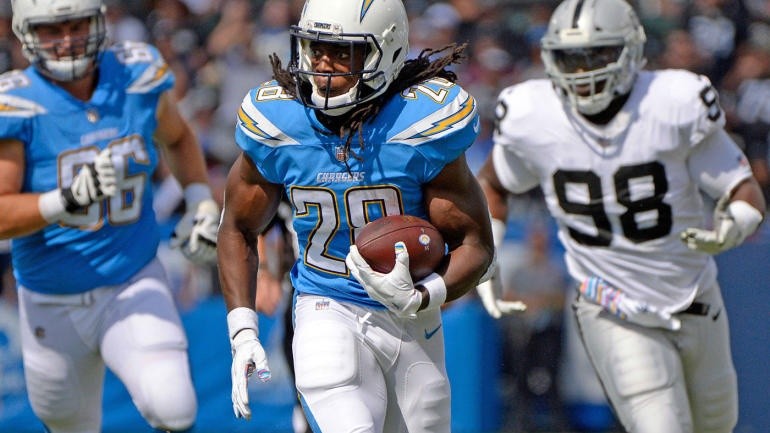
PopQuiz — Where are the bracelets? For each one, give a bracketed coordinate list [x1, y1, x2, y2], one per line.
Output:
[413, 273, 448, 312]
[38, 188, 67, 224]
[490, 216, 506, 247]
[730, 200, 761, 245]
[227, 306, 260, 340]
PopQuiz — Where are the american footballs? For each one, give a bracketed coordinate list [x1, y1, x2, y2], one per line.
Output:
[354, 213, 448, 285]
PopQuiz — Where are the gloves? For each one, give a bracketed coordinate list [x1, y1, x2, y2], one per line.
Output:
[346, 241, 422, 320]
[476, 267, 527, 319]
[60, 149, 118, 214]
[681, 200, 763, 256]
[169, 197, 220, 265]
[231, 328, 273, 420]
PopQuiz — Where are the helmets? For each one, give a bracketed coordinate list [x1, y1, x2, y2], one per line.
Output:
[11, 0, 107, 82]
[289, 0, 409, 117]
[540, 0, 649, 115]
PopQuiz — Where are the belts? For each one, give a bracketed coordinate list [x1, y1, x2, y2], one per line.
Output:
[680, 302, 722, 321]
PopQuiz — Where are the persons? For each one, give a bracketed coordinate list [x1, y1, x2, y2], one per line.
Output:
[0, 0, 770, 433]
